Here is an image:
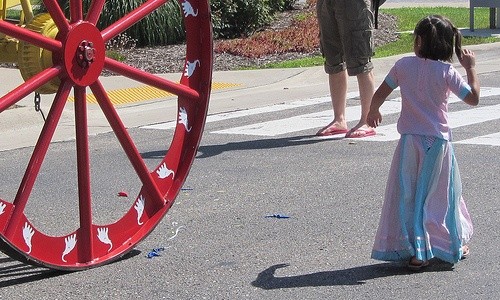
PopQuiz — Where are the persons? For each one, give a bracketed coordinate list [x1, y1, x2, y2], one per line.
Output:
[314, 0, 386, 138]
[367, 14, 481, 266]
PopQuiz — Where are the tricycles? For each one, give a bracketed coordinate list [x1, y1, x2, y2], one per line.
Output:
[0, 0, 214, 273]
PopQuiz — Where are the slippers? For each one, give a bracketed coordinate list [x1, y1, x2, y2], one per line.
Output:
[346, 127, 377, 136]
[316, 123, 349, 135]
[461, 247, 470, 259]
[406, 258, 429, 270]
